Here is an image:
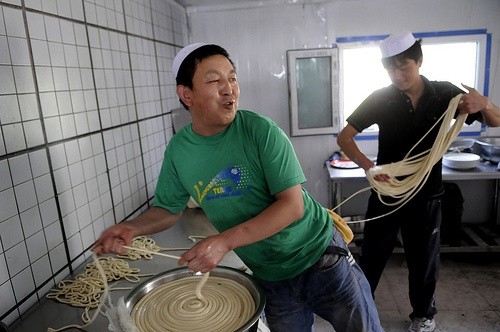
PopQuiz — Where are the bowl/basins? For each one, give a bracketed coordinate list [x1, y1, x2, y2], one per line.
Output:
[476, 136, 500, 155]
[118, 265, 267, 332]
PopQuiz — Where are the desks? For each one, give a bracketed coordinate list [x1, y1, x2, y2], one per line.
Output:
[7, 207, 261, 332]
[324, 153, 500, 255]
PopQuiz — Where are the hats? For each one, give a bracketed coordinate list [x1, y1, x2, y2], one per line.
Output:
[171, 43, 216, 80]
[377, 31, 416, 58]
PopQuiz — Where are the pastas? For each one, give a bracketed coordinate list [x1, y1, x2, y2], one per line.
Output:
[332, 93, 468, 224]
[46, 234, 255, 332]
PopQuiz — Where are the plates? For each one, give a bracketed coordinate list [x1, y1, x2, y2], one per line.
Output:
[330, 158, 360, 169]
[441, 152, 480, 169]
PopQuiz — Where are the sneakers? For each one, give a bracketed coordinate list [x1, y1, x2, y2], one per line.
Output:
[407, 317, 436, 332]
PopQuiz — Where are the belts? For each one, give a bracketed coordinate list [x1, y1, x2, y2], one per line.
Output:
[322, 246, 348, 256]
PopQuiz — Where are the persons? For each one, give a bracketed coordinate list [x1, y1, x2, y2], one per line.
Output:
[336, 29, 500, 332]
[90, 41, 387, 332]
